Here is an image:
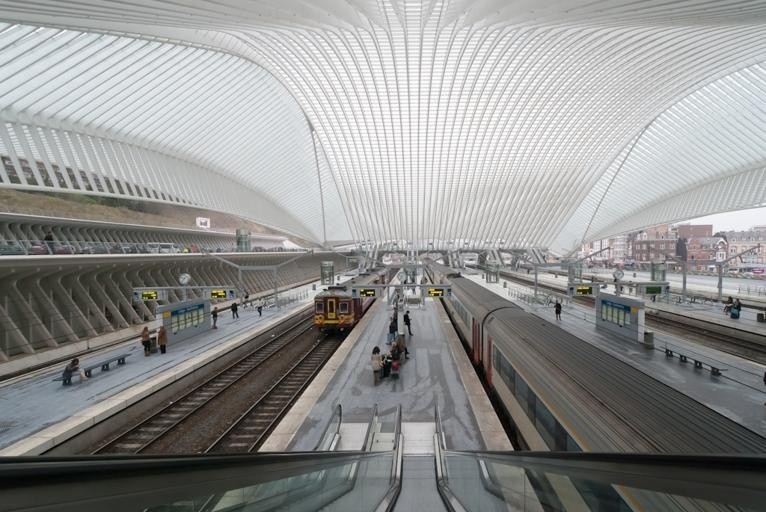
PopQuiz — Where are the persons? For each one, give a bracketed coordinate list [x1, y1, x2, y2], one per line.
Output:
[63, 358, 88, 381]
[258, 303, 263, 319]
[211, 307, 217, 329]
[727, 298, 741, 313]
[231, 303, 239, 319]
[554, 300, 562, 321]
[142, 326, 150, 357]
[158, 326, 167, 354]
[371, 307, 413, 385]
[723, 296, 734, 315]
[44, 231, 56, 254]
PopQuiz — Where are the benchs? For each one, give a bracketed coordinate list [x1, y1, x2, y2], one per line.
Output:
[713, 302, 761, 314]
[660, 343, 728, 376]
[52, 346, 135, 386]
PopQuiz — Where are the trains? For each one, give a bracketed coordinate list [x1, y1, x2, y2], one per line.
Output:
[312, 261, 401, 333]
[421, 257, 766, 511]
